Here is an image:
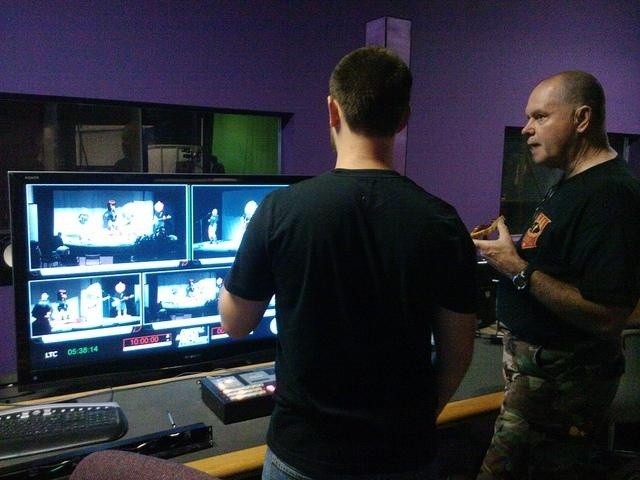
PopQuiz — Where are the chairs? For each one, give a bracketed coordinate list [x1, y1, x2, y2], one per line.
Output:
[600, 329, 640, 458]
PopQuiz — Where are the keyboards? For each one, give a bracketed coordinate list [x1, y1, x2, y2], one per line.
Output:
[0, 400, 128, 460]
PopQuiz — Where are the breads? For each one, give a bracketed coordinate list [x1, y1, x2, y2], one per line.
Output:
[470, 216, 506, 238]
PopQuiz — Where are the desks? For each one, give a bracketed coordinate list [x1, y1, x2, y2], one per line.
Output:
[0, 328, 508, 480]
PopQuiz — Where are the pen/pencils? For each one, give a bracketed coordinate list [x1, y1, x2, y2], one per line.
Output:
[167, 409, 176, 428]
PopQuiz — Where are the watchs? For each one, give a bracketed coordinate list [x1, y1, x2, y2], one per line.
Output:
[513, 264, 536, 291]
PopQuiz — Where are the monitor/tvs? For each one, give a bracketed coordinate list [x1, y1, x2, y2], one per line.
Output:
[7, 168, 319, 393]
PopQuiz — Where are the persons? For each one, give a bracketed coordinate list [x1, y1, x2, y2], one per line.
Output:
[207, 208, 219, 244]
[152, 211, 166, 229]
[112, 292, 127, 316]
[471, 70, 640, 480]
[58, 296, 70, 319]
[217, 44, 482, 480]
[36, 127, 65, 171]
[32, 292, 52, 335]
